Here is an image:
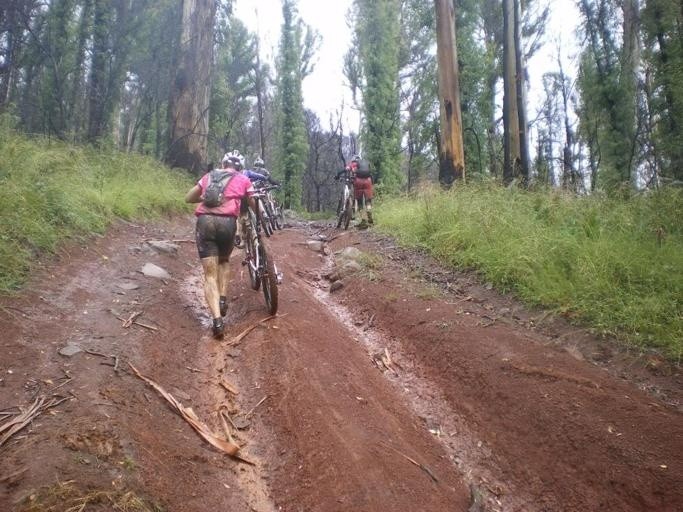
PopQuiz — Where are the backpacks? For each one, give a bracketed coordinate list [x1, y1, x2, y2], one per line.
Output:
[204, 170, 233, 207]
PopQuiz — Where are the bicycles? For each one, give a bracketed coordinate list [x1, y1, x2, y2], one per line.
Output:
[334, 174, 358, 229]
[235, 186, 284, 317]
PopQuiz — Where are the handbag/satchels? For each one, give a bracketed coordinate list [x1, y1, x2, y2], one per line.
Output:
[354, 159, 370, 177]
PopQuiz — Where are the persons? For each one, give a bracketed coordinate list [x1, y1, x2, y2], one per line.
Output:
[334, 154, 376, 226]
[184, 149, 271, 341]
[234, 167, 266, 249]
[251, 154, 282, 193]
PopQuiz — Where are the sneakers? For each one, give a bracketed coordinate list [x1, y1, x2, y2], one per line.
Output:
[354, 220, 365, 227]
[366, 210, 373, 223]
[221, 304, 228, 316]
[214, 323, 224, 336]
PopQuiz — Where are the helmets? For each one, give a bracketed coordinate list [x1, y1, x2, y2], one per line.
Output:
[222, 150, 245, 168]
[254, 158, 264, 165]
